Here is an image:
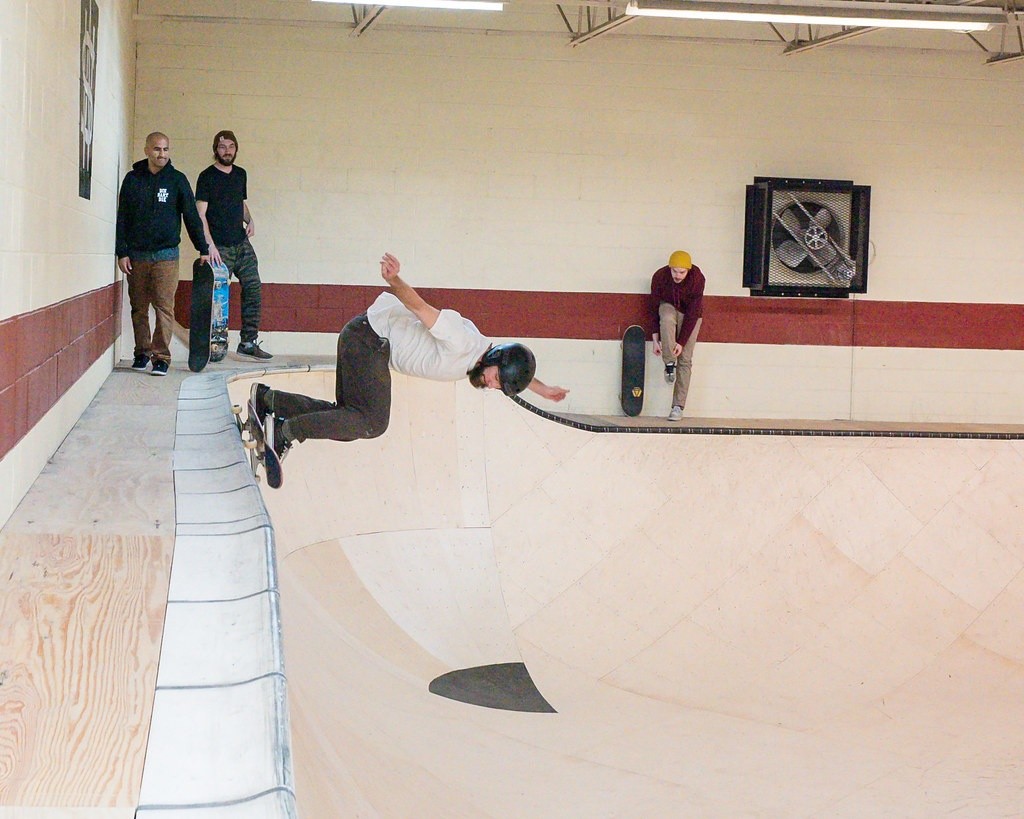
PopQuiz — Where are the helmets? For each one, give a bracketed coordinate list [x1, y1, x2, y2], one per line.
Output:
[500, 342, 536, 396]
[669, 251, 692, 269]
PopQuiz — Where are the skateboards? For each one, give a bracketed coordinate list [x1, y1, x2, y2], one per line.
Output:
[209, 261, 229, 362]
[188, 258, 215, 373]
[618, 325, 645, 418]
[231, 399, 283, 489]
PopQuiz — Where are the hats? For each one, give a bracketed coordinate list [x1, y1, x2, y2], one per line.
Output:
[213, 130, 239, 153]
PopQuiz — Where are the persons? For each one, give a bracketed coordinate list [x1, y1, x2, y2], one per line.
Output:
[250, 253, 569, 466]
[195, 130, 274, 360]
[117, 131, 210, 376]
[650, 251, 705, 421]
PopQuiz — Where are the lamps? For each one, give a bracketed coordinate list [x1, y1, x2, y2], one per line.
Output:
[624, 0, 1009, 32]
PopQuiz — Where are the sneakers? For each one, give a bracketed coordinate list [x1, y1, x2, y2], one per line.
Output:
[264, 416, 292, 464]
[250, 382, 273, 425]
[668, 406, 683, 420]
[664, 362, 676, 384]
[237, 339, 273, 360]
[131, 356, 149, 370]
[151, 361, 167, 376]
